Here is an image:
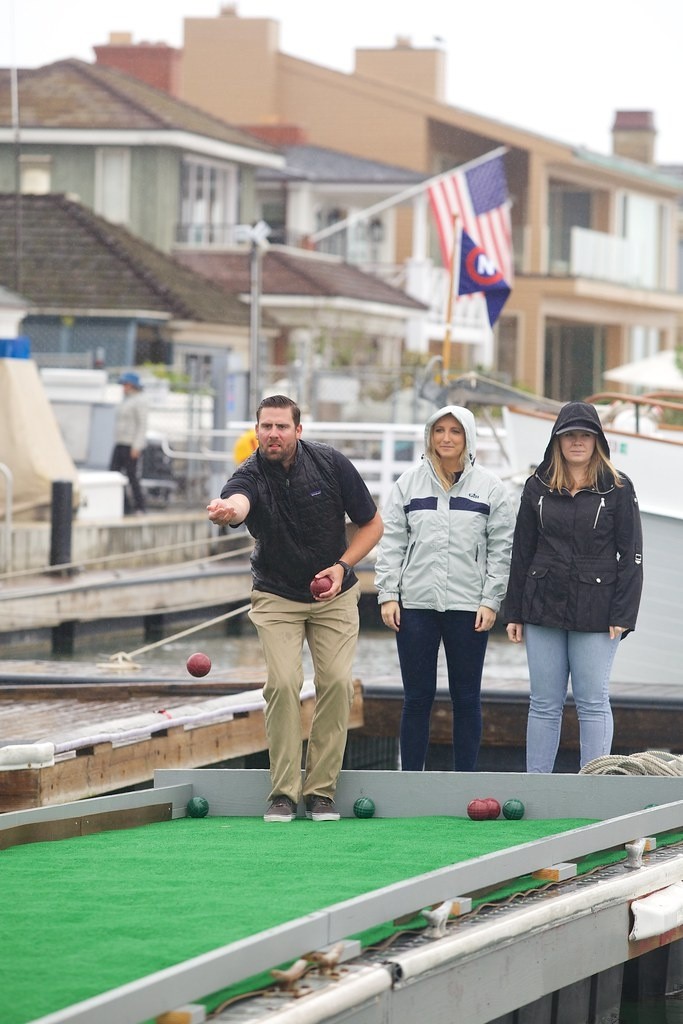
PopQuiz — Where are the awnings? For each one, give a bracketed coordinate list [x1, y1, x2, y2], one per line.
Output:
[429, 157, 513, 301]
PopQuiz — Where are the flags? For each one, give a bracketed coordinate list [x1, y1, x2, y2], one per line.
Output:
[456, 227, 512, 327]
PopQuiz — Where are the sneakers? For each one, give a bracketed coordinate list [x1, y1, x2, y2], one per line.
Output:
[263, 795, 298, 822]
[304, 795, 340, 821]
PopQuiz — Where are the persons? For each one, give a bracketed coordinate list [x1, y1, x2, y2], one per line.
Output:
[109, 373, 148, 515]
[206, 395, 384, 822]
[502, 401, 643, 774]
[374, 405, 517, 772]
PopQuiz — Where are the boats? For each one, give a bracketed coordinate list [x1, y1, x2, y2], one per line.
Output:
[227, 421, 500, 606]
[0, 334, 128, 555]
[500, 390, 682, 696]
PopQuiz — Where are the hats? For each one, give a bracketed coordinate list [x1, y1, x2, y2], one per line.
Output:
[117, 372, 142, 389]
[555, 420, 600, 435]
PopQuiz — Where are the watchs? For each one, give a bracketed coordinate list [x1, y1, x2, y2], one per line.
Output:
[333, 561, 351, 577]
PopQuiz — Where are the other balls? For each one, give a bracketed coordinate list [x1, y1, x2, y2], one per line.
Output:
[353, 796, 376, 819]
[187, 796, 210, 818]
[310, 575, 333, 597]
[185, 652, 213, 678]
[466, 797, 502, 820]
[502, 798, 526, 821]
